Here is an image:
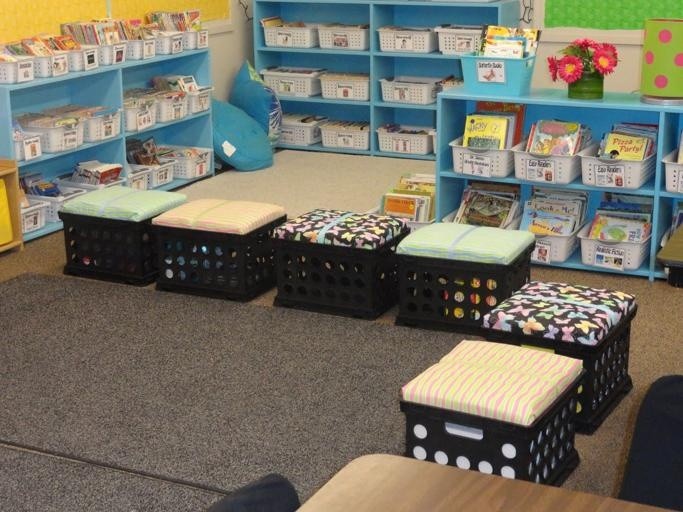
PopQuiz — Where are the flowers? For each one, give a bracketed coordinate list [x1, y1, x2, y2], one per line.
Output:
[544, 37, 621, 84]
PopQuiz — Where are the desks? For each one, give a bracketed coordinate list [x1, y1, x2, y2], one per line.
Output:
[289, 452, 683, 512]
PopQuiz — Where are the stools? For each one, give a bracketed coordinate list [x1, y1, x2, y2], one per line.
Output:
[477, 280, 640, 436]
[265, 207, 413, 322]
[399, 338, 586, 488]
[55, 184, 189, 286]
[148, 195, 288, 303]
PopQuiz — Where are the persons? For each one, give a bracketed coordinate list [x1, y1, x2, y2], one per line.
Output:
[0, 36, 62, 66]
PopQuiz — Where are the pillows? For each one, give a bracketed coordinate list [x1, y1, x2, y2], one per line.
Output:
[211, 99, 276, 172]
[227, 58, 283, 156]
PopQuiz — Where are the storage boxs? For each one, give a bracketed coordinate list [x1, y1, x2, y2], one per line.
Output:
[257, 66, 328, 98]
[316, 25, 370, 51]
[373, 123, 433, 156]
[316, 72, 371, 102]
[318, 122, 371, 152]
[31, 52, 71, 78]
[12, 113, 87, 155]
[661, 147, 683, 193]
[259, 22, 317, 49]
[575, 143, 656, 190]
[124, 35, 157, 60]
[19, 198, 51, 236]
[377, 75, 444, 108]
[24, 184, 88, 225]
[532, 219, 593, 263]
[96, 40, 129, 66]
[575, 217, 652, 269]
[279, 118, 320, 147]
[153, 144, 214, 180]
[146, 158, 180, 189]
[54, 174, 128, 193]
[154, 30, 185, 56]
[0, 55, 36, 85]
[39, 106, 125, 144]
[66, 46, 99, 73]
[12, 129, 44, 162]
[457, 51, 538, 98]
[509, 140, 582, 186]
[375, 27, 438, 54]
[184, 28, 210, 51]
[128, 165, 153, 192]
[433, 25, 488, 55]
[439, 206, 524, 231]
[447, 133, 514, 179]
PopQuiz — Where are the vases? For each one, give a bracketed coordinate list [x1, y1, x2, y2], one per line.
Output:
[567, 71, 605, 101]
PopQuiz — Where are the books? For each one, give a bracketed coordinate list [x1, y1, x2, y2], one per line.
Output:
[146, 10, 211, 51]
[588, 200, 655, 241]
[123, 138, 162, 166]
[528, 116, 591, 153]
[17, 160, 128, 215]
[125, 73, 211, 110]
[374, 171, 435, 222]
[519, 188, 589, 238]
[458, 104, 525, 150]
[9, 104, 121, 143]
[450, 182, 520, 237]
[595, 119, 659, 162]
[479, 25, 542, 56]
[63, 19, 145, 58]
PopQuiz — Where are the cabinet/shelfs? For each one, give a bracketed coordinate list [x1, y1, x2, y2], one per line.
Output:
[0, 46, 218, 246]
[433, 83, 682, 283]
[253, 0, 521, 165]
